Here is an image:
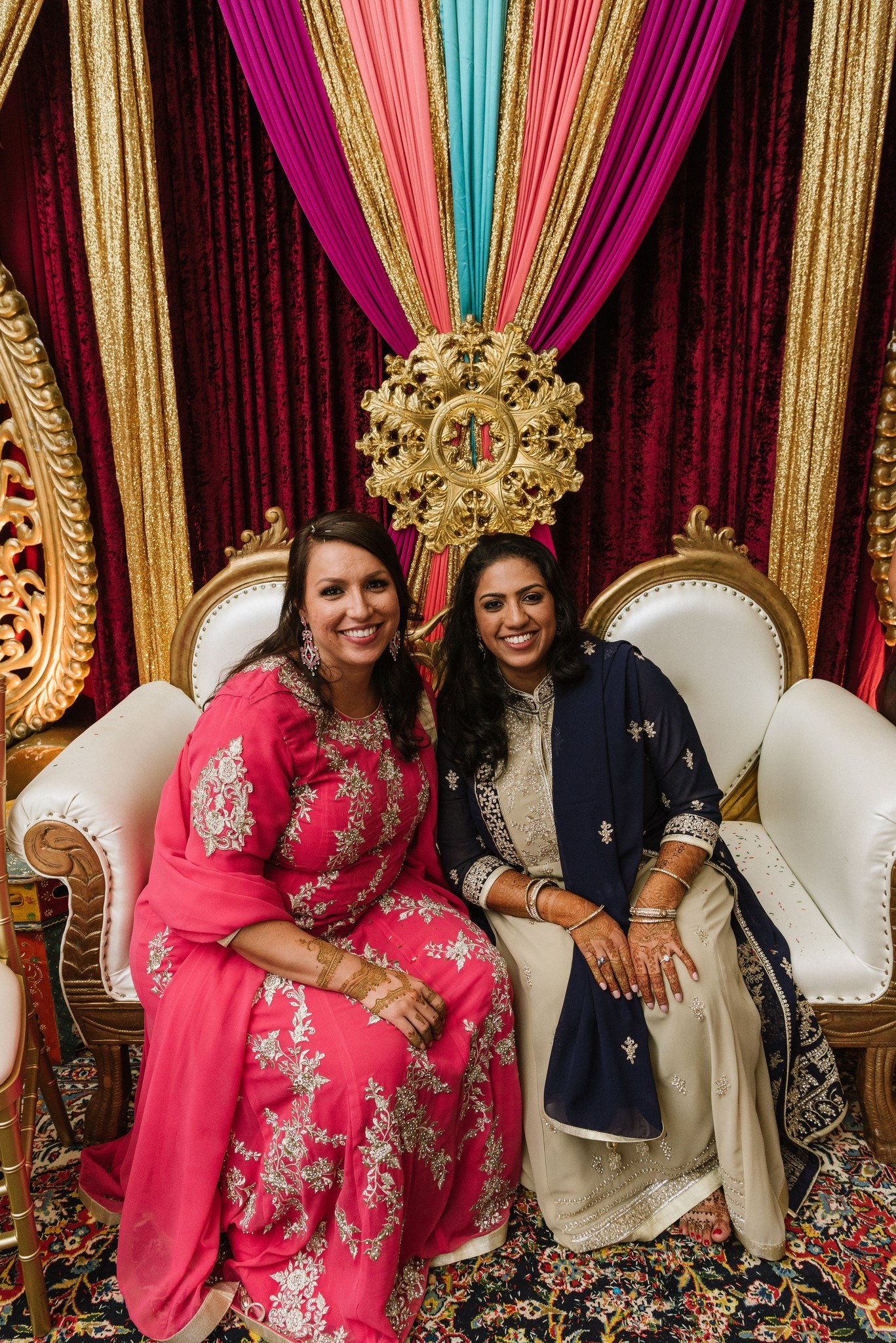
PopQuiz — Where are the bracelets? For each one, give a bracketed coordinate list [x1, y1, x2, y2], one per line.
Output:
[627, 916, 675, 923]
[529, 877, 560, 923]
[524, 878, 543, 921]
[649, 867, 691, 890]
[565, 903, 605, 934]
[629, 905, 677, 918]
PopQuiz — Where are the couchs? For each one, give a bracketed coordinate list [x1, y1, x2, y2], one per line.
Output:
[14, 510, 896, 1169]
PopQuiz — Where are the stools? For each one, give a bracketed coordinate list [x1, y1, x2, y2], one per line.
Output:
[3, 962, 79, 1334]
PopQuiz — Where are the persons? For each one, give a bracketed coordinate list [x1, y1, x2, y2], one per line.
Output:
[83, 508, 521, 1343]
[436, 531, 851, 1259]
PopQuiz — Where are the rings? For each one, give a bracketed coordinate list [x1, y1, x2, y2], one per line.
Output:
[660, 955, 673, 963]
[597, 955, 610, 968]
